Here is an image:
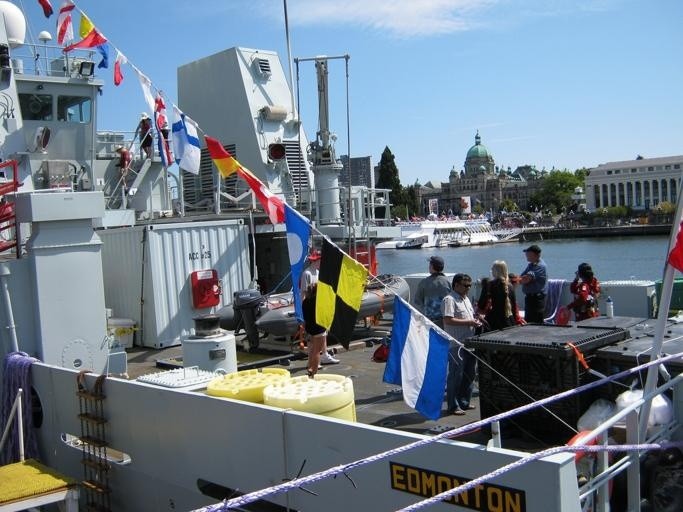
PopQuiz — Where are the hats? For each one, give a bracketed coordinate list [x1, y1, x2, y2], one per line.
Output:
[307, 250, 321, 261]
[426, 255, 444, 270]
[114, 145, 122, 152]
[522, 245, 541, 253]
[140, 113, 148, 120]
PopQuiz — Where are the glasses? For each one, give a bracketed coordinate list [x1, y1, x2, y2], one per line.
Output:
[458, 283, 471, 288]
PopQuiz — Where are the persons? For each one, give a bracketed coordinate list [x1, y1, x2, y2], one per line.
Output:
[566, 262, 601, 322]
[519, 244, 549, 324]
[300, 250, 341, 369]
[302, 285, 326, 378]
[440, 273, 481, 415]
[414, 255, 452, 331]
[477, 259, 527, 335]
[135, 112, 153, 159]
[114, 144, 132, 193]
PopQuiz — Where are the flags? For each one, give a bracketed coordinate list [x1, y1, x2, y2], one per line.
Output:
[56, 0, 76, 46]
[38, 0, 55, 19]
[171, 103, 201, 176]
[96, 41, 110, 69]
[238, 165, 285, 226]
[152, 91, 172, 168]
[205, 136, 241, 180]
[79, 13, 94, 40]
[137, 73, 155, 112]
[62, 28, 107, 53]
[282, 202, 311, 326]
[314, 234, 369, 351]
[382, 295, 451, 421]
[113, 51, 128, 87]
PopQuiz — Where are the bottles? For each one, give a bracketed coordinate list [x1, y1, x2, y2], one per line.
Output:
[606, 296, 613, 319]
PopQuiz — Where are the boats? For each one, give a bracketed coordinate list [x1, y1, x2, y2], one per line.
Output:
[374, 219, 514, 252]
[0, 1, 683, 511]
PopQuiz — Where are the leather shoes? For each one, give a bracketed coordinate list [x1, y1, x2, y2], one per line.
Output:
[306, 364, 323, 370]
[321, 357, 341, 364]
